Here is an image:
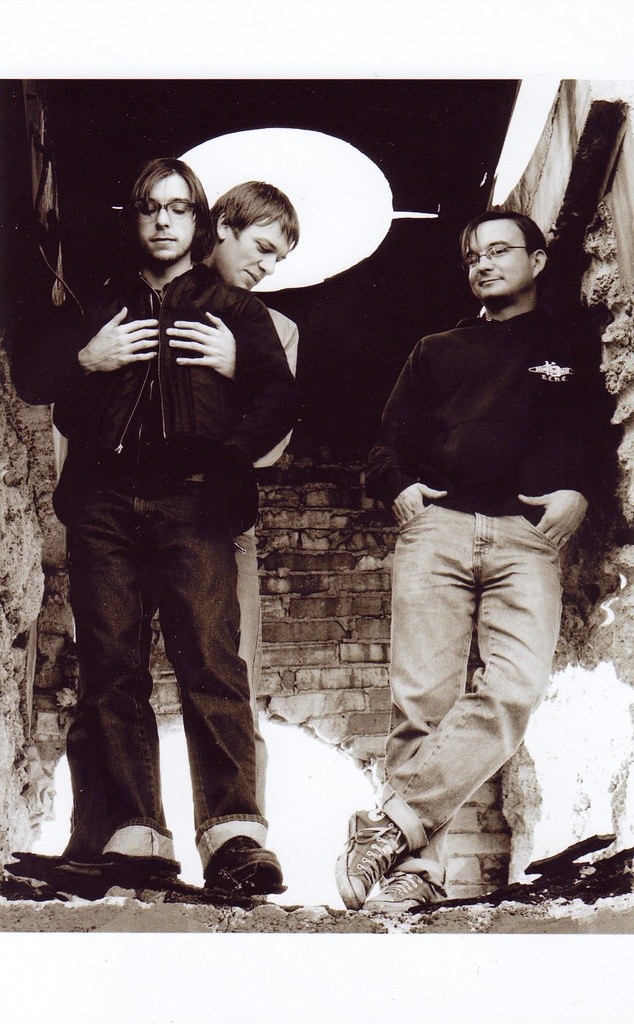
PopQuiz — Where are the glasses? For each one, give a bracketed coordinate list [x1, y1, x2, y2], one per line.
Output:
[135, 200, 195, 219]
[462, 245, 531, 271]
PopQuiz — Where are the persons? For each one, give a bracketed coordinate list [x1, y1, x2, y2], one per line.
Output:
[9, 153, 302, 898]
[335, 208, 622, 917]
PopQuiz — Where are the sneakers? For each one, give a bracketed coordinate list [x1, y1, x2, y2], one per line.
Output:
[363, 867, 439, 913]
[335, 809, 409, 911]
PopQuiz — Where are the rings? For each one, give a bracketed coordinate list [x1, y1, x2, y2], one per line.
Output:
[561, 537, 568, 542]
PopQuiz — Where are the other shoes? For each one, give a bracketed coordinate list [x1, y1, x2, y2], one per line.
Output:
[50, 850, 181, 901]
[204, 836, 283, 898]
